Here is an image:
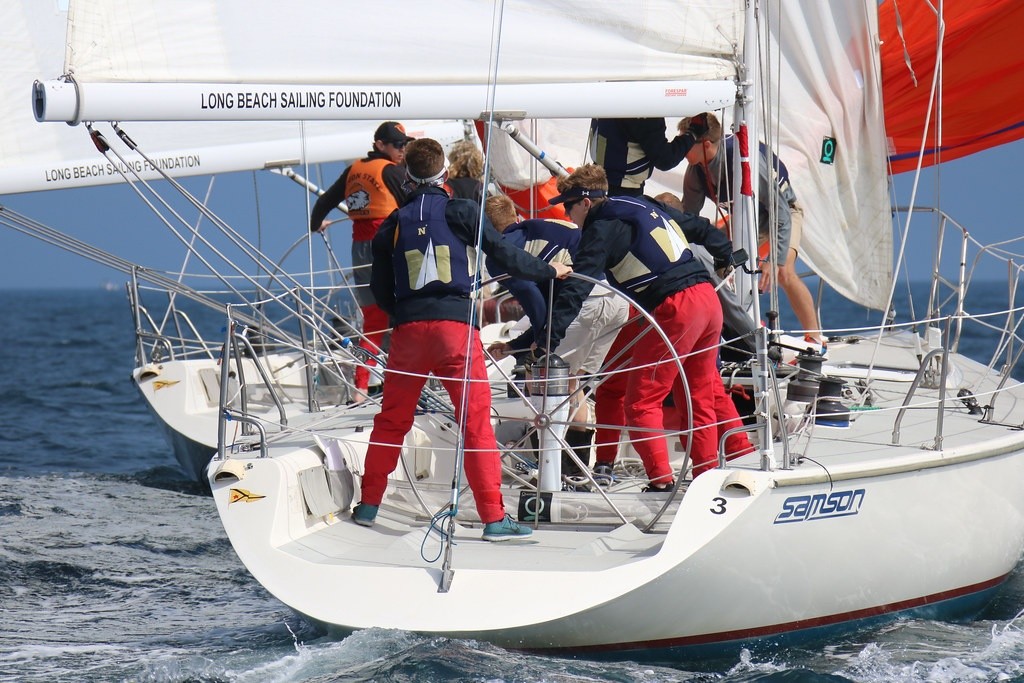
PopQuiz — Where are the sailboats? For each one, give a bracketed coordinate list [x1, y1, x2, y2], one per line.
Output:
[0, 0, 1024, 659]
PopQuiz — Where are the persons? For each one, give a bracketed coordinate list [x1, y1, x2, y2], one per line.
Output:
[654, 113, 827, 366]
[479, 166, 757, 492]
[311, 121, 415, 408]
[446, 140, 492, 204]
[589, 112, 709, 197]
[351, 138, 573, 542]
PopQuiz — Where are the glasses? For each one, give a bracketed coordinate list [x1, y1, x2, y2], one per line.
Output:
[385, 139, 408, 149]
[563, 199, 583, 213]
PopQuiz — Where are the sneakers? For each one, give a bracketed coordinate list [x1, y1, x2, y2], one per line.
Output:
[351, 501, 378, 525]
[788, 333, 828, 366]
[482, 512, 532, 541]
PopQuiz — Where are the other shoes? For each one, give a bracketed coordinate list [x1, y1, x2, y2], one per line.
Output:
[642, 478, 674, 493]
[346, 400, 366, 407]
[592, 462, 613, 485]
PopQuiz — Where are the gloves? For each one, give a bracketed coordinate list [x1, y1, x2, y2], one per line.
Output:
[686, 111, 710, 141]
[511, 346, 553, 378]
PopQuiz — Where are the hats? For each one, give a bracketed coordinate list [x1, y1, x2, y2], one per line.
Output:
[548, 185, 607, 205]
[374, 121, 415, 144]
[406, 155, 450, 184]
